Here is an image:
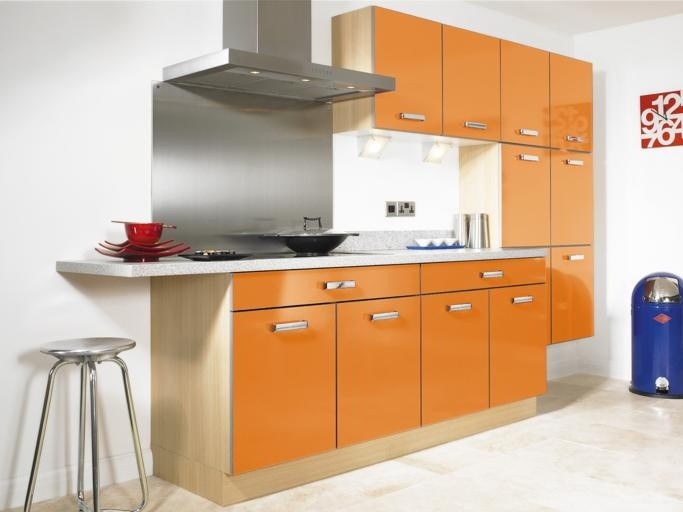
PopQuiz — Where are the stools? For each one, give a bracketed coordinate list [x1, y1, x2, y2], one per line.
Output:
[25, 336, 149, 511]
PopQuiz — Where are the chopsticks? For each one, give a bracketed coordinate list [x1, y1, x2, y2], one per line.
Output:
[110, 220, 177, 230]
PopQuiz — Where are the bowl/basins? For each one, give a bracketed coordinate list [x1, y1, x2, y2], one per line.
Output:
[122, 222, 163, 245]
[414, 238, 458, 247]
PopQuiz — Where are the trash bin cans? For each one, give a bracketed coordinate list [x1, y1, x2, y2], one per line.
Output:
[629, 271, 683, 399]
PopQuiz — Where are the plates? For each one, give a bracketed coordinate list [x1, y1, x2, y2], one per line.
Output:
[176, 253, 252, 260]
[93, 240, 193, 262]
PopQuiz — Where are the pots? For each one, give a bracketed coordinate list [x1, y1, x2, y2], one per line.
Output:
[262, 214, 360, 254]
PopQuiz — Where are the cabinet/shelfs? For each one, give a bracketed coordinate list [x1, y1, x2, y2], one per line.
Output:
[545, 246, 596, 346]
[502, 36, 593, 152]
[149, 264, 420, 476]
[458, 145, 595, 248]
[421, 257, 547, 424]
[331, 6, 501, 147]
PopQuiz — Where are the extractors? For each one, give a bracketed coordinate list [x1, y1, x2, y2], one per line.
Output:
[160, 0, 396, 106]
